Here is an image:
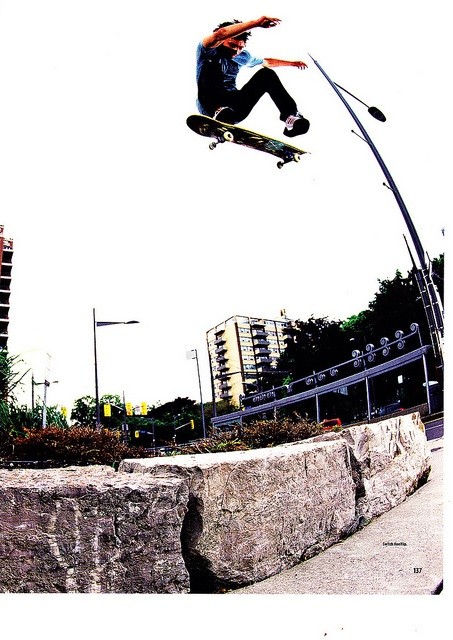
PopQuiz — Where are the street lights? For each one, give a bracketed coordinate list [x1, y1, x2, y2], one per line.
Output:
[327, 77, 428, 272]
[32, 379, 59, 422]
[91, 307, 138, 429]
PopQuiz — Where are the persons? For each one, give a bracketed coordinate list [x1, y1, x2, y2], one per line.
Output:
[194, 14, 311, 137]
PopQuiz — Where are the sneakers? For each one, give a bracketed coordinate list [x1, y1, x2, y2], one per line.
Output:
[213, 107, 236, 125]
[283, 112, 310, 137]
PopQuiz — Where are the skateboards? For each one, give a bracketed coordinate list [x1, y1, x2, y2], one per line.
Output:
[186, 114, 311, 169]
